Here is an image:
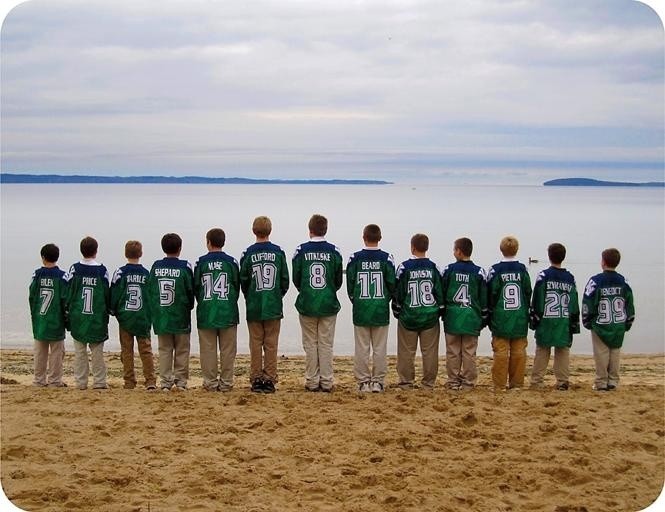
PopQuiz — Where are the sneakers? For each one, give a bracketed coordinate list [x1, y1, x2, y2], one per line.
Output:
[250, 378, 382, 393]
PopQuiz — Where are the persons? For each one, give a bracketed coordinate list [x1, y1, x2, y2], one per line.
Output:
[346, 223, 398, 393]
[147, 233, 193, 390]
[392, 233, 443, 388]
[193, 227, 240, 392]
[582, 247, 636, 391]
[62, 235, 112, 389]
[486, 234, 533, 389]
[442, 236, 488, 391]
[110, 240, 156, 389]
[28, 243, 68, 387]
[240, 215, 289, 394]
[527, 242, 581, 389]
[292, 214, 343, 393]
